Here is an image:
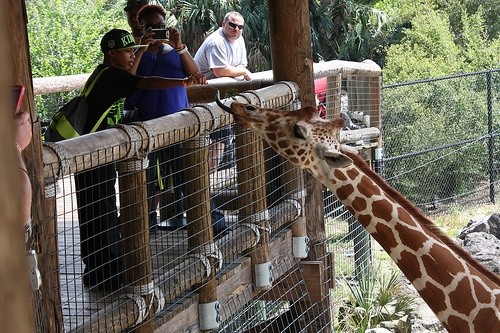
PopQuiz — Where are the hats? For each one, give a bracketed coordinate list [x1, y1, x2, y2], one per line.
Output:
[124, 0, 149, 12]
[100, 28, 149, 53]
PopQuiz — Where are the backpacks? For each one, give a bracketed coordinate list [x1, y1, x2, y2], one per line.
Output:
[44, 67, 126, 142]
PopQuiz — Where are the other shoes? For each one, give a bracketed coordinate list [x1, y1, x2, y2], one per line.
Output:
[83, 266, 126, 292]
[214, 227, 232, 239]
[118, 212, 159, 234]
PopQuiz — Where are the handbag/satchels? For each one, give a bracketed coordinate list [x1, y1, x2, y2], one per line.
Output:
[117, 104, 146, 125]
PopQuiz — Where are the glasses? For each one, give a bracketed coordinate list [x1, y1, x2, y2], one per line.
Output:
[224, 21, 244, 29]
[140, 23, 165, 32]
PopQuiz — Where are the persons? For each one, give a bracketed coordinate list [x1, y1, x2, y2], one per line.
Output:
[73, 29, 209, 288]
[124, 5, 230, 238]
[194, 10, 253, 174]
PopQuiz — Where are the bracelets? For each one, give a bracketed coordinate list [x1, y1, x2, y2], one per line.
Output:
[183, 78, 188, 88]
[175, 44, 188, 54]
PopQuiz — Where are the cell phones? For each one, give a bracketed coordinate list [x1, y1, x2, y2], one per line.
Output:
[8, 84, 25, 116]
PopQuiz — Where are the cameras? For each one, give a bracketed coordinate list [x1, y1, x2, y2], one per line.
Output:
[150, 29, 169, 41]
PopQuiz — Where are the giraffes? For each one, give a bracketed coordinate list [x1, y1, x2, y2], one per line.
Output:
[229, 100, 500, 333]
